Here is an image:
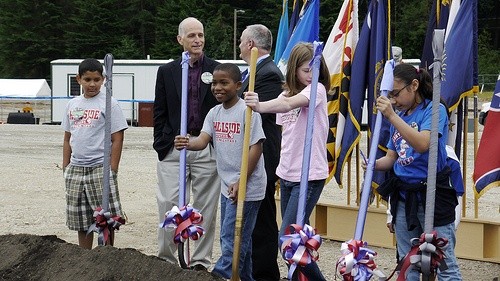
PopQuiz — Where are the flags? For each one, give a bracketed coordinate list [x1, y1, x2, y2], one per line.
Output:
[473, 74, 500, 199]
[418, 0, 479, 113]
[322, 0, 392, 207]
[274, 0, 320, 78]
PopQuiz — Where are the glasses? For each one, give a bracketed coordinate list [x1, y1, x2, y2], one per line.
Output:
[387, 84, 411, 98]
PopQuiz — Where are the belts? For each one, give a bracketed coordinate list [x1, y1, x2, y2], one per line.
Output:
[176, 129, 201, 137]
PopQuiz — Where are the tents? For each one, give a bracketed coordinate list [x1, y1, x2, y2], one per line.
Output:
[0, 78, 52, 116]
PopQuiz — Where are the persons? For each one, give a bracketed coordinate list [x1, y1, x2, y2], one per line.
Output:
[236, 24, 286, 281]
[244, 41, 331, 281]
[392, 46, 404, 64]
[61, 59, 128, 250]
[362, 63, 461, 281]
[174, 63, 267, 281]
[152, 16, 223, 274]
